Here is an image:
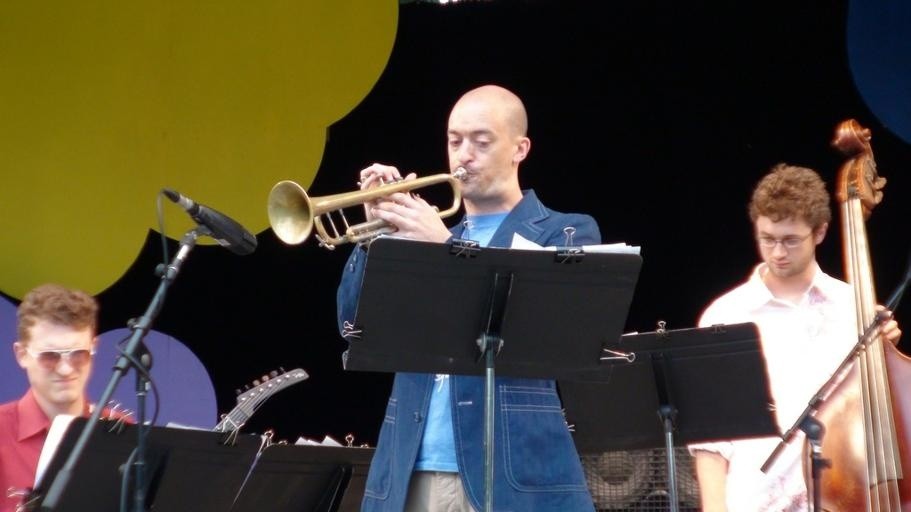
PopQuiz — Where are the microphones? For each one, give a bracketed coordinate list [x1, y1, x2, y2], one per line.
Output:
[158, 187, 259, 256]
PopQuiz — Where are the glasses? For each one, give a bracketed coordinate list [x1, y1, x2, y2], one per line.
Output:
[756, 230, 813, 249]
[24, 344, 96, 372]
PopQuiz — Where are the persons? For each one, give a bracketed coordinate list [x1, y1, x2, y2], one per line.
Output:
[335, 83, 602, 512]
[0, 284, 137, 511]
[685, 163, 904, 511]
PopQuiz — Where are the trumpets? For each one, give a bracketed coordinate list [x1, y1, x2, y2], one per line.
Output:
[266, 166, 468, 249]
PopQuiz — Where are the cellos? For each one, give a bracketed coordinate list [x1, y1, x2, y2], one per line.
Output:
[801, 120, 911, 509]
